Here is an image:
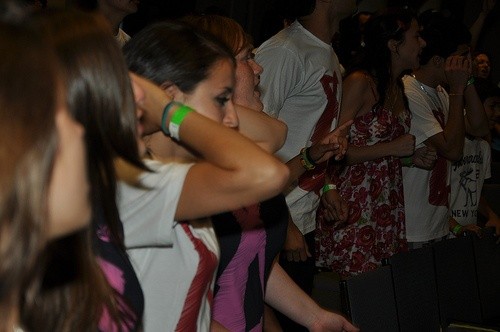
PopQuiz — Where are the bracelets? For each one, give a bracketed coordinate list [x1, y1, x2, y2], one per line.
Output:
[161, 101, 184, 135]
[453, 224, 461, 236]
[300, 147, 316, 170]
[466, 77, 476, 86]
[168, 105, 196, 140]
[319, 184, 338, 196]
[404, 156, 412, 166]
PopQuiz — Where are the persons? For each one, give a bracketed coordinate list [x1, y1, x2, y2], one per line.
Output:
[0, 26, 89, 332]
[23, 0, 500, 332]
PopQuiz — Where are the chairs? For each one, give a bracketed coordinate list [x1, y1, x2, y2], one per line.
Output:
[340, 231, 500, 332]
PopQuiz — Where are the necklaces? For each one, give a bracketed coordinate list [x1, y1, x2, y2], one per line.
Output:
[385, 85, 400, 109]
[410, 73, 443, 110]
